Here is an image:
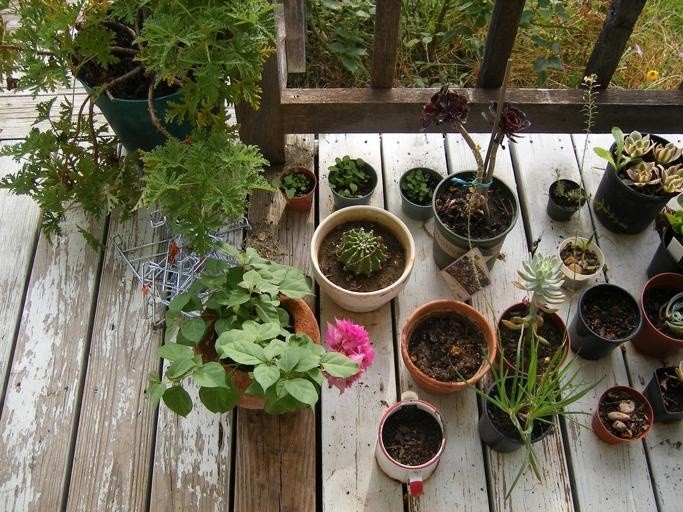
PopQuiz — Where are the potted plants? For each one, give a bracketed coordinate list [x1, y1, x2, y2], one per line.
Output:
[490, 253, 570, 378]
[646, 194, 683, 285]
[143, 237, 370, 416]
[0, 0, 285, 258]
[450, 314, 609, 501]
[569, 284, 642, 357]
[420, 58, 567, 382]
[400, 166, 443, 221]
[642, 361, 681, 430]
[311, 205, 415, 313]
[328, 156, 376, 210]
[557, 235, 604, 293]
[401, 298, 498, 395]
[626, 273, 682, 357]
[280, 167, 317, 212]
[591, 386, 654, 445]
[545, 71, 629, 230]
[374, 391, 449, 498]
[593, 126, 683, 234]
